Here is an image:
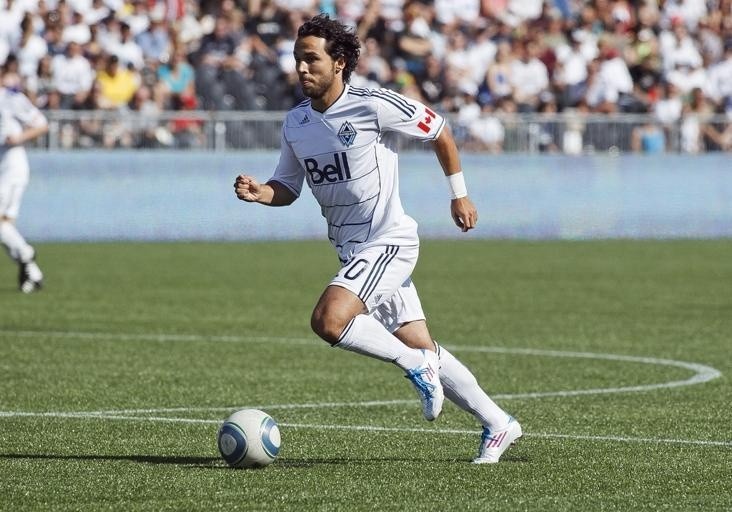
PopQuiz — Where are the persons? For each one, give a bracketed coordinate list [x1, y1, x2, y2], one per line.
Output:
[1, 1, 732, 152]
[0, 88, 42, 294]
[234, 15, 522, 465]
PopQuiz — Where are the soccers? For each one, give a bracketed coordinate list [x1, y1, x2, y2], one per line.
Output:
[218, 408, 282, 469]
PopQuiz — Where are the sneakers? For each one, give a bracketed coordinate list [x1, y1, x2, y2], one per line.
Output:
[404, 350, 445, 421]
[469, 417, 522, 464]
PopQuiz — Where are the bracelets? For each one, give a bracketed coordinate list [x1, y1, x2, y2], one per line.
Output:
[444, 171, 468, 200]
